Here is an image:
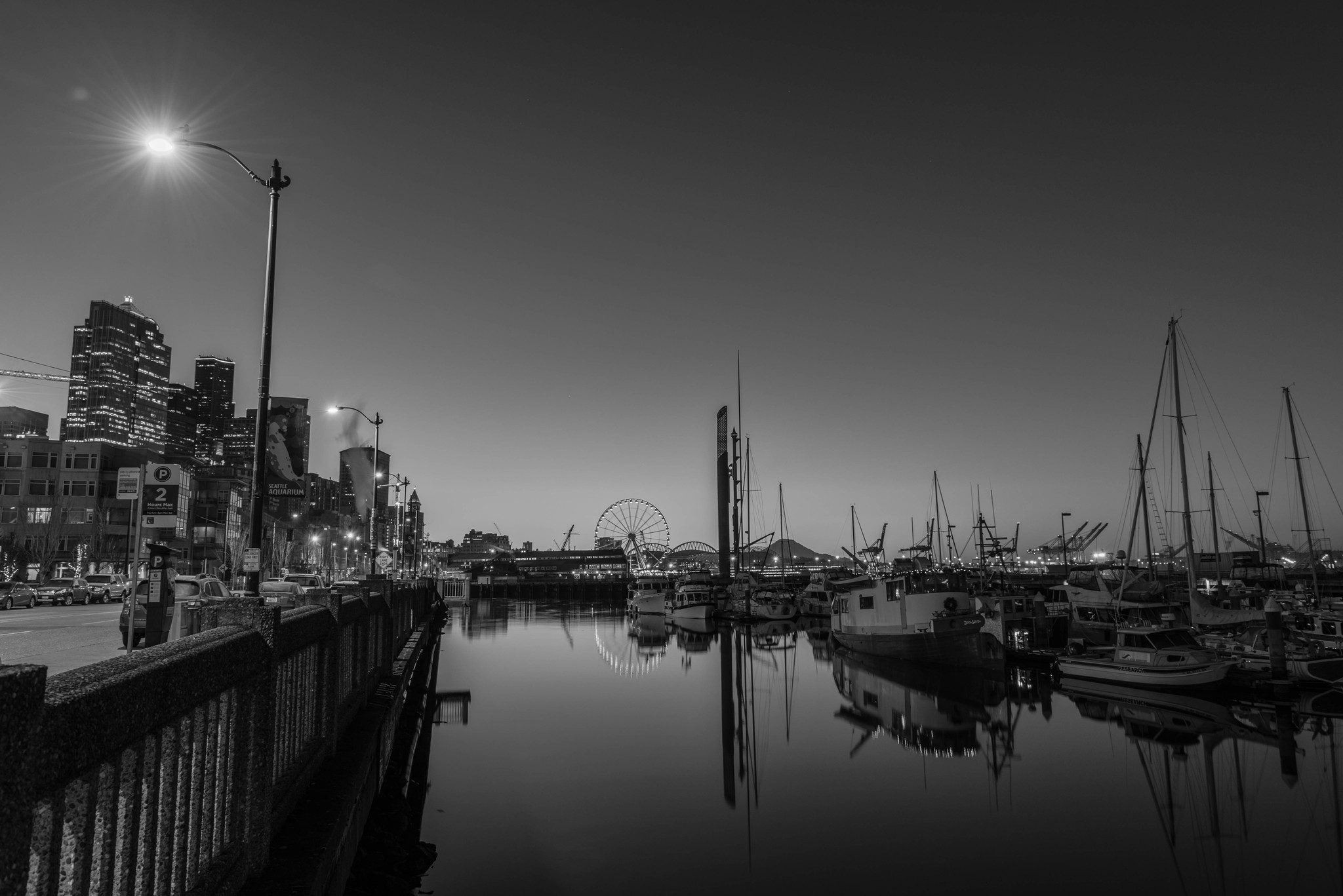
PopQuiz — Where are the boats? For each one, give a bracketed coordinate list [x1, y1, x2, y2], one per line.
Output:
[628, 608, 669, 664]
[665, 567, 716, 619]
[626, 568, 668, 614]
[665, 613, 718, 676]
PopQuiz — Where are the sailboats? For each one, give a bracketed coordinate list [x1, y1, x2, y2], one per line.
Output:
[730, 619, 1343, 896]
[727, 315, 1343, 687]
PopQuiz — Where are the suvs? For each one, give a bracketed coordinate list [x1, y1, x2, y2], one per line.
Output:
[35, 577, 92, 606]
[119, 573, 240, 648]
[85, 573, 129, 604]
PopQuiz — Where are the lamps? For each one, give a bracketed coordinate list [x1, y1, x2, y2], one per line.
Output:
[3, 445, 7, 448]
[70, 447, 75, 450]
[21, 446, 26, 449]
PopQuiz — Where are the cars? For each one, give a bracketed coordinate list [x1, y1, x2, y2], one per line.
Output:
[266, 574, 366, 594]
[0, 582, 38, 610]
[258, 582, 305, 612]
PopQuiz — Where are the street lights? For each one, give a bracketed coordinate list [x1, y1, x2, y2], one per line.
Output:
[376, 473, 410, 580]
[142, 130, 290, 606]
[414, 530, 430, 575]
[406, 519, 426, 577]
[328, 405, 387, 580]
[419, 538, 439, 579]
[395, 502, 420, 579]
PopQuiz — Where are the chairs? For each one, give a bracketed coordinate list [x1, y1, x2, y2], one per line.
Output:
[309, 580, 315, 585]
[291, 580, 296, 582]
